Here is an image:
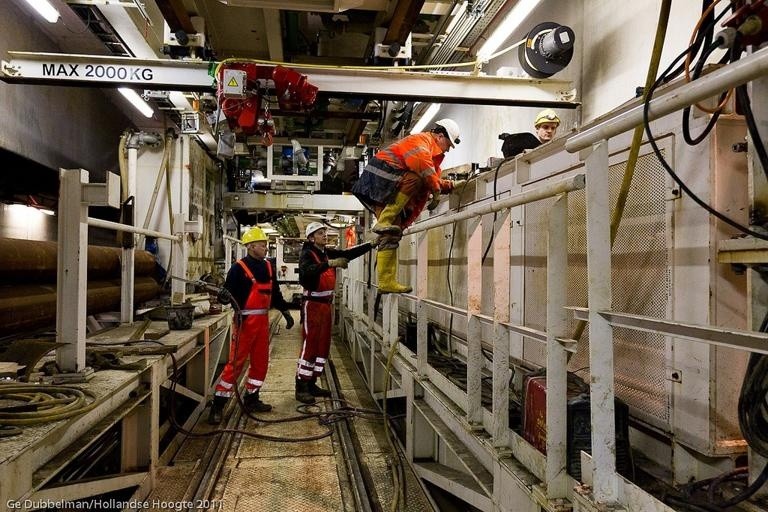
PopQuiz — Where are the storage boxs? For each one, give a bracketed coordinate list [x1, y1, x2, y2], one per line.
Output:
[521, 373, 636, 483]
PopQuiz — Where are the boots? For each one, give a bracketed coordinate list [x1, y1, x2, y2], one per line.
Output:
[243, 388, 272, 411]
[371, 191, 409, 234]
[376, 248, 412, 294]
[295, 379, 316, 404]
[207, 395, 230, 426]
[314, 384, 331, 397]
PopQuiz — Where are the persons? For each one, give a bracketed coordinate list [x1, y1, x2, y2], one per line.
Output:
[295, 221, 379, 404]
[208, 226, 293, 425]
[535, 109, 560, 144]
[352, 119, 458, 293]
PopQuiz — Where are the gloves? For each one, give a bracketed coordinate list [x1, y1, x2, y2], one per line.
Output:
[370, 235, 386, 249]
[217, 288, 231, 306]
[327, 257, 348, 269]
[281, 310, 294, 330]
[452, 179, 467, 188]
[427, 192, 441, 210]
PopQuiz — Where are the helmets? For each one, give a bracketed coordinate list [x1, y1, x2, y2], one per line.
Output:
[306, 221, 326, 238]
[241, 227, 268, 246]
[435, 118, 460, 149]
[534, 109, 561, 129]
